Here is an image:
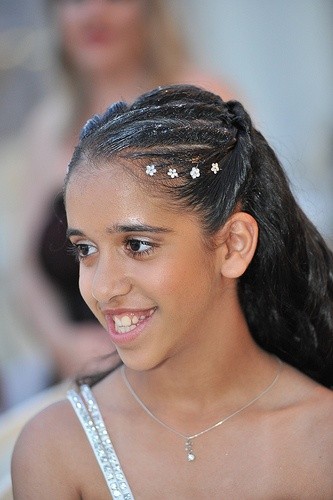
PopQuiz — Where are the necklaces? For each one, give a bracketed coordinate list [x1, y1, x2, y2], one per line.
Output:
[117, 362, 290, 464]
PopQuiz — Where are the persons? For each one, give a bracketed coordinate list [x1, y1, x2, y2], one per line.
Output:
[0, 0, 254, 455]
[9, 85, 333, 500]
